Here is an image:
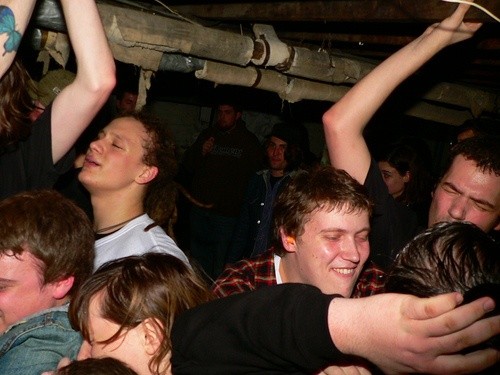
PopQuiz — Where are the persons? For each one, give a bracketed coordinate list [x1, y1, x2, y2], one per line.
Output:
[0, 70, 500, 375]
[322, 0, 500, 272]
[0, 0, 117, 200]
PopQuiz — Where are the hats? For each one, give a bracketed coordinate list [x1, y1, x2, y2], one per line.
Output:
[263, 120, 308, 144]
